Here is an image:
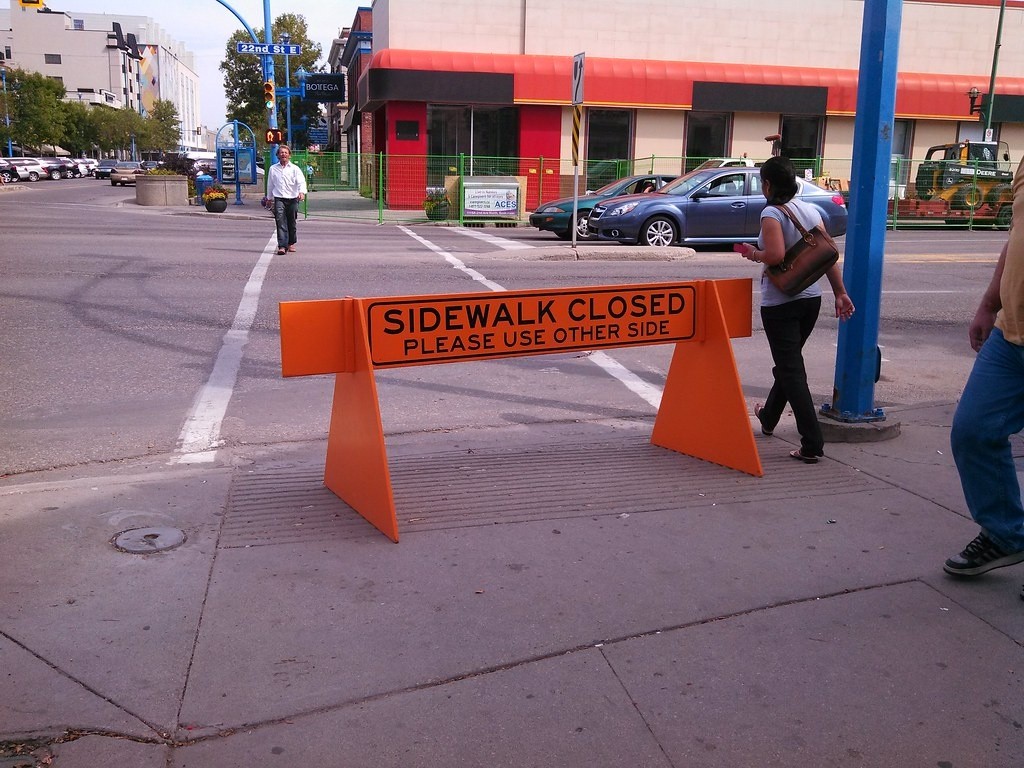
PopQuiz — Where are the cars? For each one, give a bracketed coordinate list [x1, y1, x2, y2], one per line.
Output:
[4, 157, 50, 182]
[255, 156, 266, 179]
[141, 160, 165, 172]
[94, 159, 121, 179]
[0, 158, 18, 183]
[528, 174, 681, 241]
[33, 157, 100, 181]
[182, 158, 218, 177]
[110, 161, 146, 186]
[587, 166, 848, 246]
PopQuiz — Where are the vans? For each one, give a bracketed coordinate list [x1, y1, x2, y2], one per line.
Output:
[685, 158, 766, 193]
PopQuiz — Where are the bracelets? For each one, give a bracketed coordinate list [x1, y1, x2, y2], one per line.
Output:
[753, 249, 761, 263]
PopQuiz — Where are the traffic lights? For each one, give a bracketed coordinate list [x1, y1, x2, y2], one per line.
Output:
[265, 128, 282, 144]
[264, 82, 276, 112]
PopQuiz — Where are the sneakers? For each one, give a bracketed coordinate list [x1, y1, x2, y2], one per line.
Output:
[944, 528, 1024, 576]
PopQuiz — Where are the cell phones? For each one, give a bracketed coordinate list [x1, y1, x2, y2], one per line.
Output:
[733, 244, 746, 252]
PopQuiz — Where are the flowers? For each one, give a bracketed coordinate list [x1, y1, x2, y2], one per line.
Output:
[421, 188, 452, 214]
[201, 183, 229, 206]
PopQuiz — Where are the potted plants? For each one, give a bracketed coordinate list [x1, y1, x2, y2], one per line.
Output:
[136, 167, 189, 206]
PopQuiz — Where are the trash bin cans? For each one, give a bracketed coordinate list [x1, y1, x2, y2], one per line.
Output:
[195, 175, 214, 205]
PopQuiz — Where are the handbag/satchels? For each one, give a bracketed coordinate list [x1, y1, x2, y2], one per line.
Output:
[759, 204, 841, 300]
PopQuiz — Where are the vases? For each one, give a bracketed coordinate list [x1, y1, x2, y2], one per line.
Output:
[426, 204, 451, 221]
[204, 199, 228, 213]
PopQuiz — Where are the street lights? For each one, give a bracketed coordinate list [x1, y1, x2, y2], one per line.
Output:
[277, 29, 310, 148]
[967, 0, 1009, 143]
[130, 133, 137, 162]
[1, 68, 13, 158]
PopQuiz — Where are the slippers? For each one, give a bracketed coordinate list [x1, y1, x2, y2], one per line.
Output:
[789, 448, 818, 465]
[756, 402, 774, 434]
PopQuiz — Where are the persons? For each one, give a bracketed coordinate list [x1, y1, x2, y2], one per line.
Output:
[725, 180, 736, 194]
[267, 145, 307, 255]
[506, 191, 515, 200]
[943, 155, 1024, 600]
[306, 163, 317, 192]
[742, 156, 856, 464]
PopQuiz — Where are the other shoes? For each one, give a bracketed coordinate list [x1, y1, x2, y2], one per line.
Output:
[279, 247, 286, 255]
[288, 244, 296, 252]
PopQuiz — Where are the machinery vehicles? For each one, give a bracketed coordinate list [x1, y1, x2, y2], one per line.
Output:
[888, 139, 1015, 230]
